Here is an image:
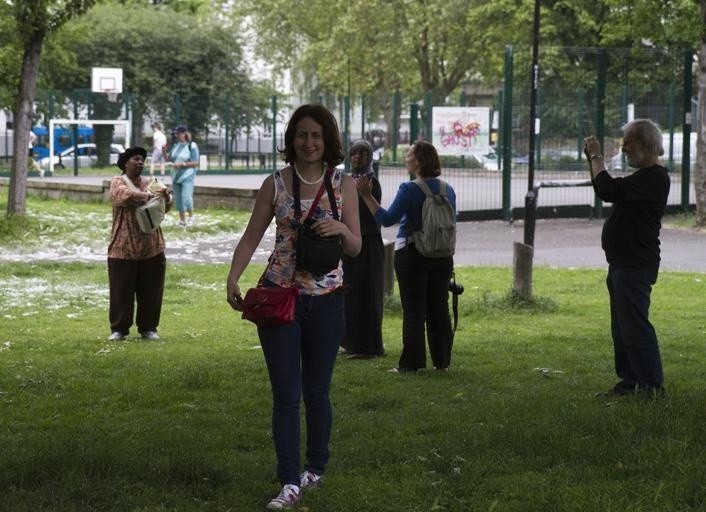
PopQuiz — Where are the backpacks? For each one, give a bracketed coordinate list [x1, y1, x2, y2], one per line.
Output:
[412, 194, 458, 260]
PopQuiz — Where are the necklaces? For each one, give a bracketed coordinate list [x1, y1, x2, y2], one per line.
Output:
[293, 162, 327, 185]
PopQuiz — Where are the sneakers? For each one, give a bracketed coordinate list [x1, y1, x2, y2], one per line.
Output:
[140, 331, 161, 341]
[106, 331, 128, 342]
[177, 219, 186, 227]
[264, 481, 301, 511]
[385, 365, 428, 376]
[187, 218, 195, 228]
[298, 466, 325, 490]
[592, 388, 666, 403]
[432, 365, 450, 377]
[335, 346, 378, 361]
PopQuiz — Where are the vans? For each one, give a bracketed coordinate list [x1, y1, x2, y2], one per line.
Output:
[609, 130, 698, 173]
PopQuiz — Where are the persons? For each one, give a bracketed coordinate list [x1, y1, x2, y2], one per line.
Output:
[357, 140, 456, 374]
[149, 122, 167, 176]
[584, 118, 671, 407]
[108, 147, 174, 342]
[226, 105, 363, 510]
[28, 128, 50, 178]
[337, 140, 386, 360]
[162, 126, 200, 227]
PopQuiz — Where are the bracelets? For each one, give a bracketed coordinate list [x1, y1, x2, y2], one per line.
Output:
[591, 153, 603, 160]
[183, 162, 185, 167]
[166, 194, 172, 205]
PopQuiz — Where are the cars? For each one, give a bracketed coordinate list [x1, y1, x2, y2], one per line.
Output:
[37, 143, 129, 168]
[371, 145, 586, 171]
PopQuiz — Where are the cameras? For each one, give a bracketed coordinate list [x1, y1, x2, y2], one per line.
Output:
[449, 282, 464, 295]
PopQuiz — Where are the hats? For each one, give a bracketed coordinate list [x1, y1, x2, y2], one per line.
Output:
[116, 146, 148, 171]
[171, 124, 189, 134]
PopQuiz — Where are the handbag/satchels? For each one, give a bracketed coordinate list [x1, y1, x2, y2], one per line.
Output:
[240, 286, 300, 324]
[292, 229, 342, 277]
[134, 195, 167, 234]
